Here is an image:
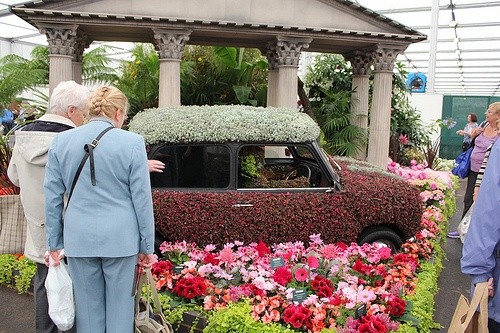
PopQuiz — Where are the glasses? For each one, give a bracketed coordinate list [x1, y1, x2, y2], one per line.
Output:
[255, 154, 265, 158]
[485, 108, 497, 114]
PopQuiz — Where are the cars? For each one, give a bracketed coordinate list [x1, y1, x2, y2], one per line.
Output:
[124, 105, 424, 261]
[0, 108, 15, 135]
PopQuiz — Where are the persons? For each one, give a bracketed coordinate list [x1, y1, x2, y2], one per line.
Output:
[447, 101, 500, 333]
[7, 81, 165, 333]
[45, 86, 154, 333]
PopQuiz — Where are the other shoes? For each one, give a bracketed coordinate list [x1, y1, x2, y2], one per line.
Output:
[448, 231, 460, 239]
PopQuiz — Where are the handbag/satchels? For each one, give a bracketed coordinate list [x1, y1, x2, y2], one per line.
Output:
[135, 268, 174, 333]
[447, 282, 489, 333]
[452, 138, 475, 179]
[45, 249, 75, 331]
